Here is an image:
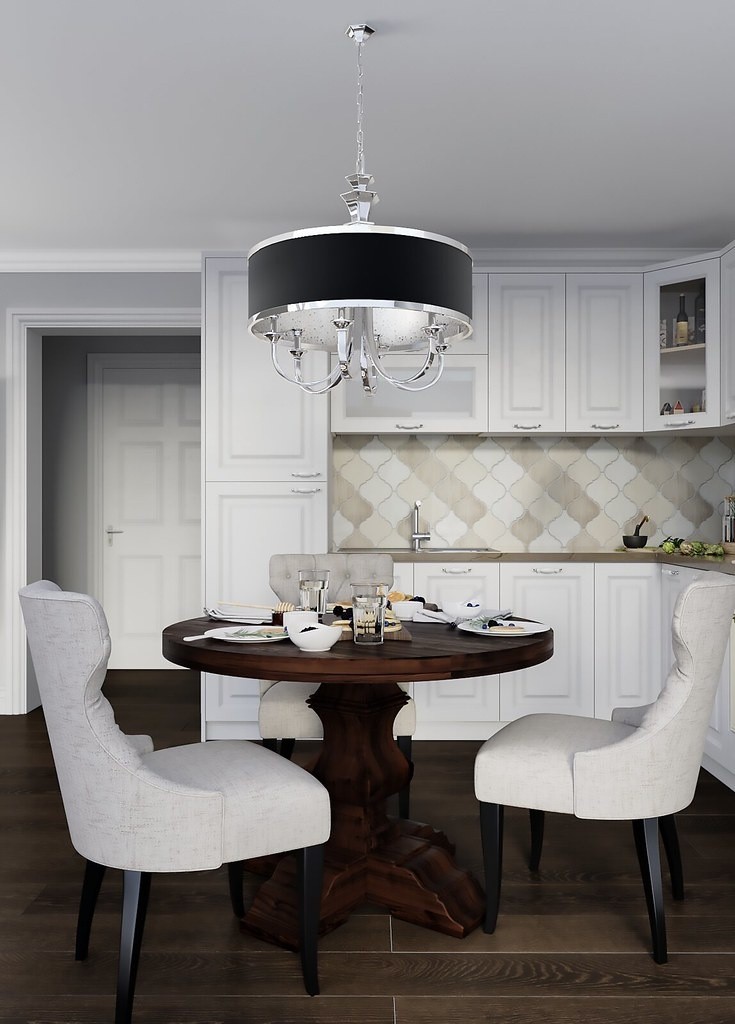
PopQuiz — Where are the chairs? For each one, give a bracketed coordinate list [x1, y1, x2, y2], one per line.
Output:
[256, 553, 418, 820]
[13, 575, 336, 1024]
[477, 575, 735, 964]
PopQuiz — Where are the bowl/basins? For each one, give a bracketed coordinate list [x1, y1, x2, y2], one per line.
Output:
[283, 611, 318, 628]
[623, 536, 648, 548]
[393, 601, 423, 619]
[289, 628, 343, 652]
[441, 604, 481, 618]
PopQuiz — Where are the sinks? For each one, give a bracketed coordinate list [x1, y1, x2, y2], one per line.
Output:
[335, 547, 413, 553]
[424, 547, 495, 554]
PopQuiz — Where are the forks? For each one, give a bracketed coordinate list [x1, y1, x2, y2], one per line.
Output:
[183, 626, 248, 642]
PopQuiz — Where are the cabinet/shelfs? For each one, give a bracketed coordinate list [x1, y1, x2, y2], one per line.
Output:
[198, 240, 735, 794]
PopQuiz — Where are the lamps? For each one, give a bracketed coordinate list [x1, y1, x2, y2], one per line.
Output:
[234, 18, 479, 401]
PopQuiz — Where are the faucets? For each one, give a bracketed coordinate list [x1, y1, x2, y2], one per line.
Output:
[410, 500, 431, 552]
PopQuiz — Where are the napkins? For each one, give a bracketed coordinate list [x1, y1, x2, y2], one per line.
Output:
[414, 604, 514, 628]
[197, 603, 274, 625]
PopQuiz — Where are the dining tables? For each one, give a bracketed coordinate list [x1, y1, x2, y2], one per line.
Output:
[159, 605, 553, 951]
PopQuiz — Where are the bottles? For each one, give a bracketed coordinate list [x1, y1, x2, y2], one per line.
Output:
[677, 293, 688, 345]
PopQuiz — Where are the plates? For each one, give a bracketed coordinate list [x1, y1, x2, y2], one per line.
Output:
[457, 618, 551, 636]
[205, 626, 289, 643]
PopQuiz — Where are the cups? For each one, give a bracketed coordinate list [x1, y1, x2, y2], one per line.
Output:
[350, 583, 389, 645]
[298, 570, 329, 618]
